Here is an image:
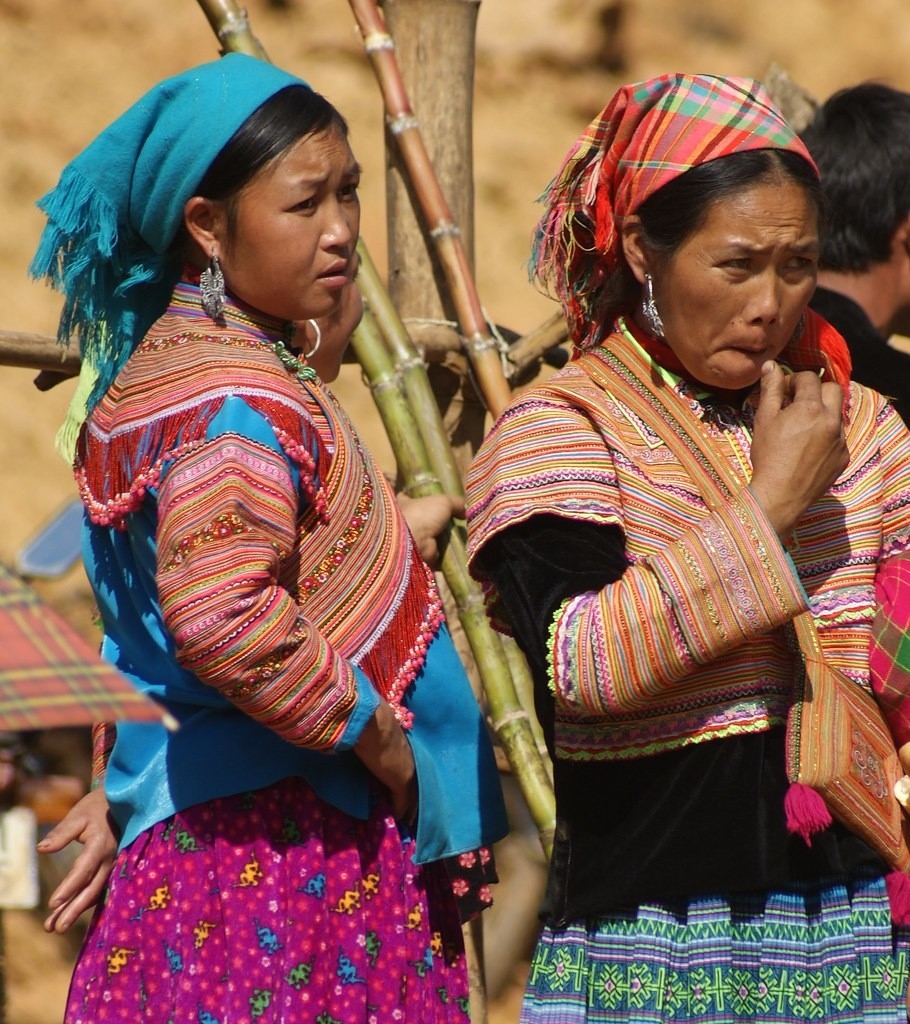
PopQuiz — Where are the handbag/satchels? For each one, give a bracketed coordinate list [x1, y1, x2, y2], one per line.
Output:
[783, 654, 910, 880]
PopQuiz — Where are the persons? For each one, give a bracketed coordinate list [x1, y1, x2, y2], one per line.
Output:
[290, 242, 469, 570]
[788, 79, 907, 432]
[29, 47, 484, 1024]
[460, 74, 908, 1024]
